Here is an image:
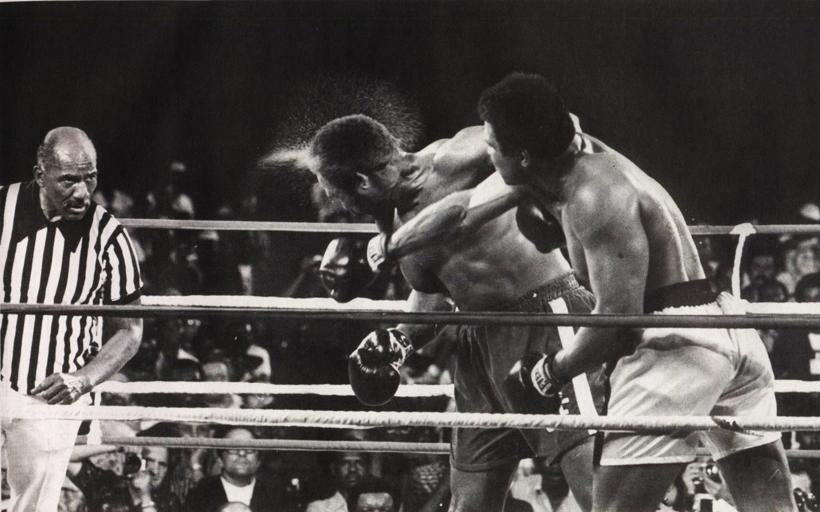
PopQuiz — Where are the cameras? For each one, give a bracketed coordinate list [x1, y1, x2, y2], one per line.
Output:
[693, 463, 719, 485]
[793, 487, 818, 509]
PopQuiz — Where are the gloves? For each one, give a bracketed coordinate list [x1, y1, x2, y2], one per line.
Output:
[320, 233, 393, 303]
[504, 352, 560, 414]
[349, 328, 415, 408]
[516, 198, 565, 254]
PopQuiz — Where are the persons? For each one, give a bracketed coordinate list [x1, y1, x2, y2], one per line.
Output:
[316, 72, 799, 512]
[306, 114, 607, 512]
[1, 125, 144, 510]
[509, 201, 819, 509]
[62, 160, 448, 511]
